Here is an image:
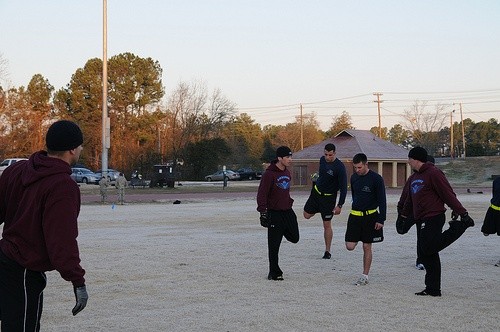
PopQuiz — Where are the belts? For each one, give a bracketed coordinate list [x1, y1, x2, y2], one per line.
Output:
[314, 185, 332, 196]
[350, 208, 377, 217]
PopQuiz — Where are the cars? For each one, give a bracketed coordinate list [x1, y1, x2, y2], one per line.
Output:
[94, 169, 125, 183]
[238, 168, 264, 180]
[70, 167, 101, 185]
[0, 157, 29, 176]
[205, 170, 241, 182]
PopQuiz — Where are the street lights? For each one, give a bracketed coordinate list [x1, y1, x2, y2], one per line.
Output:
[450, 109, 456, 159]
[138, 91, 158, 175]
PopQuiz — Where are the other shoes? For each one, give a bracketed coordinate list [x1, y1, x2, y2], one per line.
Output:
[267, 274, 284, 281]
[415, 289, 441, 296]
[494, 261, 500, 267]
[322, 250, 331, 259]
[351, 274, 369, 287]
[415, 264, 424, 270]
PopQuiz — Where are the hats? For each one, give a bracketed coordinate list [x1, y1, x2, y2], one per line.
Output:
[408, 146, 427, 162]
[427, 155, 435, 163]
[46, 120, 83, 151]
[276, 146, 293, 156]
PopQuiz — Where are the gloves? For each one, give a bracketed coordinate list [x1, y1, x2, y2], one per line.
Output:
[451, 210, 459, 220]
[484, 233, 489, 236]
[461, 212, 474, 226]
[310, 171, 320, 183]
[259, 214, 271, 228]
[398, 208, 402, 214]
[72, 286, 88, 316]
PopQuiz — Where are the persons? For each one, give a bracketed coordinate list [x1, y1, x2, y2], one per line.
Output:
[303, 143, 348, 258]
[481, 177, 500, 267]
[0, 120, 89, 332]
[344, 153, 387, 285]
[99, 176, 109, 205]
[115, 172, 128, 205]
[396, 146, 476, 296]
[257, 146, 299, 281]
